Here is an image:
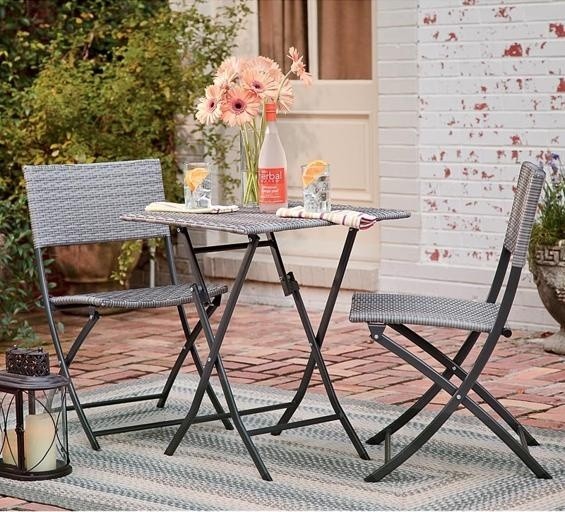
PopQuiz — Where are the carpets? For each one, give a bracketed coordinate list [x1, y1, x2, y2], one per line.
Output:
[0, 373, 564, 512]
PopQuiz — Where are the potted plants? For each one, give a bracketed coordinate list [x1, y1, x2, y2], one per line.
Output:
[527, 151, 565, 353]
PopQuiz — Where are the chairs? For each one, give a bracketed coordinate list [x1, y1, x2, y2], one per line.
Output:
[348, 161, 551, 483]
[23, 159, 234, 450]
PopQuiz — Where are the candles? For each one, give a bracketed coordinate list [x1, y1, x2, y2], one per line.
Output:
[0, 344, 72, 480]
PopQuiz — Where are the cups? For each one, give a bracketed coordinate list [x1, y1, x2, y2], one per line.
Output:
[184, 163, 211, 210]
[302, 162, 330, 213]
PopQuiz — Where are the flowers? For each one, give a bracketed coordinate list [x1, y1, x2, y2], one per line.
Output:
[195, 47, 314, 129]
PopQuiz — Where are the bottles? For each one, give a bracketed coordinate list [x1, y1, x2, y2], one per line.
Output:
[258, 104, 288, 213]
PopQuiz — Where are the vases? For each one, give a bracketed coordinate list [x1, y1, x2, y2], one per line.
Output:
[46, 239, 144, 316]
[241, 130, 266, 207]
